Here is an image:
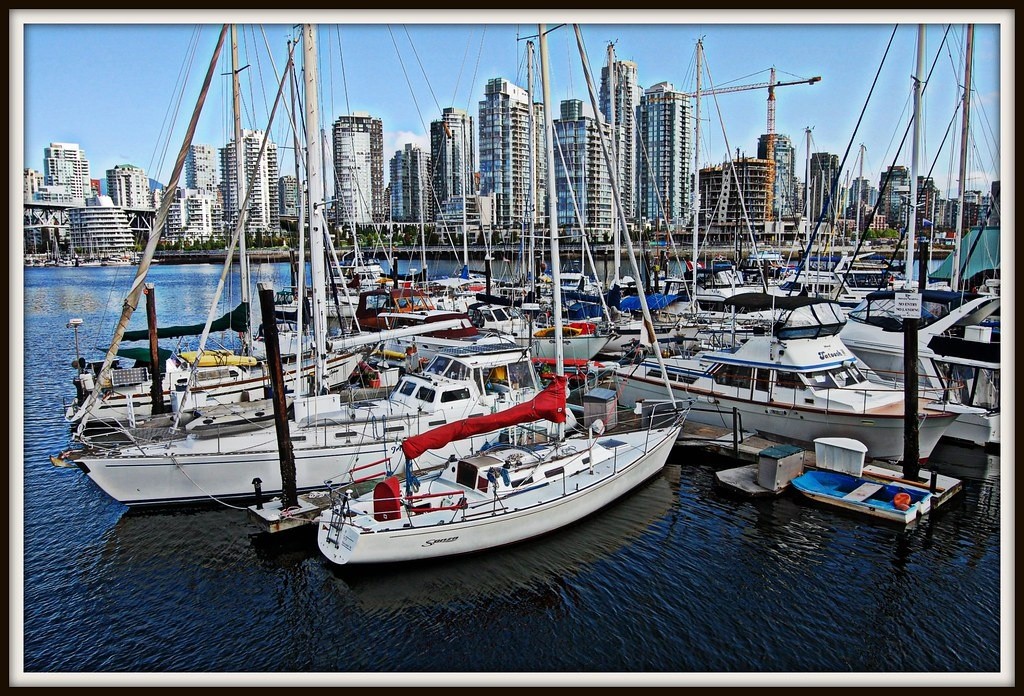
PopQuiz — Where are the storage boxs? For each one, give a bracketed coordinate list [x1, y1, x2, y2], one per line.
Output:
[378, 368, 399, 388]
[293, 394, 340, 423]
[964, 325, 992, 343]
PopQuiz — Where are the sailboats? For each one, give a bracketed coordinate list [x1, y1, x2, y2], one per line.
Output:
[50, 25, 578, 512]
[224, 24, 999, 468]
[316, 25, 691, 567]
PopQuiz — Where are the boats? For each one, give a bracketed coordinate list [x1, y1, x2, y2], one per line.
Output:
[790, 470, 932, 524]
[612, 295, 988, 476]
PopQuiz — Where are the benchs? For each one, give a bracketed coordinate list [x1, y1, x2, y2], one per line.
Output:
[843, 482, 883, 502]
